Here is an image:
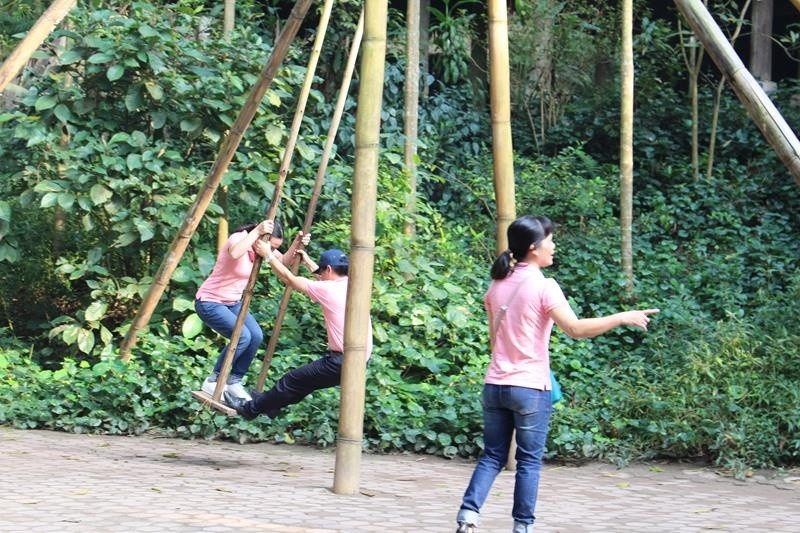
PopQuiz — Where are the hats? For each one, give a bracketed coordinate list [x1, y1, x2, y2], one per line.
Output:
[312, 249, 349, 274]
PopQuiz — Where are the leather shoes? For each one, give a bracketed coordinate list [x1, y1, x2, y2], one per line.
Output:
[224, 391, 254, 421]
[249, 388, 280, 419]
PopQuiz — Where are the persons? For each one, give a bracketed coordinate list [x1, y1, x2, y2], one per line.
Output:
[455, 217, 660, 533]
[224, 239, 374, 421]
[195, 220, 311, 403]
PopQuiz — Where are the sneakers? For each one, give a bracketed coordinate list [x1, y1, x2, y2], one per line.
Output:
[456, 522, 476, 533]
[201, 377, 228, 401]
[228, 382, 252, 401]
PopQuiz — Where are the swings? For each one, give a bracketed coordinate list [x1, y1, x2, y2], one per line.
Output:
[187, 0, 365, 420]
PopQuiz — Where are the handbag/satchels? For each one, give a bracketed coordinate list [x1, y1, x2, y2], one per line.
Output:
[549, 371, 561, 405]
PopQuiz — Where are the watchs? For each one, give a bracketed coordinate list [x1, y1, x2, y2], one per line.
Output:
[265, 253, 275, 264]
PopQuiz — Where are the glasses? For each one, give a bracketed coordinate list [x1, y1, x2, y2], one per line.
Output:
[319, 271, 323, 278]
